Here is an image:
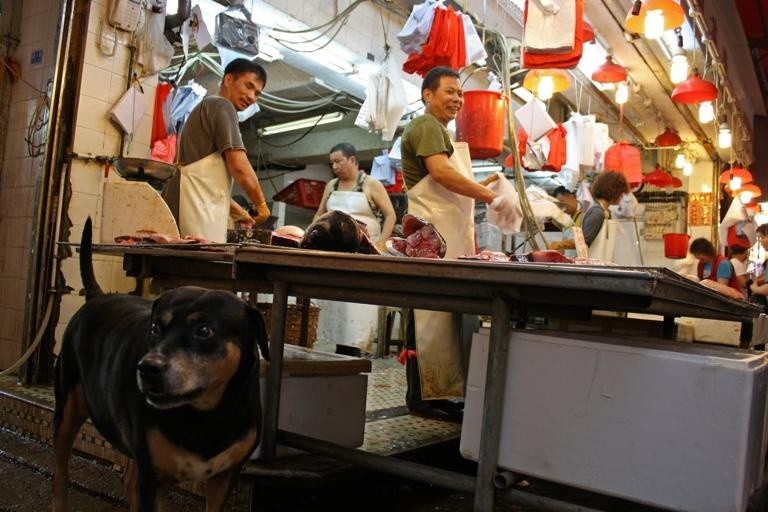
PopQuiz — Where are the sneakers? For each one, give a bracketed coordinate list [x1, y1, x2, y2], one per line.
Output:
[407, 399, 462, 422]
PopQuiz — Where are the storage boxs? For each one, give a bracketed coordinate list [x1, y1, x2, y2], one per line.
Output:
[249, 336, 374, 465]
[456, 317, 768, 511]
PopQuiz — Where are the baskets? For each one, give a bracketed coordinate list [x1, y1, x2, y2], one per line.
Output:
[260, 303, 320, 347]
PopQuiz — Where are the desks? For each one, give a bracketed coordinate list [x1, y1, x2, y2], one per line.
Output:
[116, 239, 768, 511]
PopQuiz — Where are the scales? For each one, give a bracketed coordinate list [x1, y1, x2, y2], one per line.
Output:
[100, 157, 181, 243]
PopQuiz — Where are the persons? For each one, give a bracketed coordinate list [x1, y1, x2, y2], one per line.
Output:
[162, 57, 271, 247]
[546, 187, 585, 252]
[298, 139, 397, 364]
[685, 214, 768, 294]
[395, 69, 524, 425]
[577, 170, 629, 263]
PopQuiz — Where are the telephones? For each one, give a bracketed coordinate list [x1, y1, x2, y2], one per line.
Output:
[108, 1, 142, 32]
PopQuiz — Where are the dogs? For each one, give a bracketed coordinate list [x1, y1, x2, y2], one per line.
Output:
[48, 215, 272, 512]
